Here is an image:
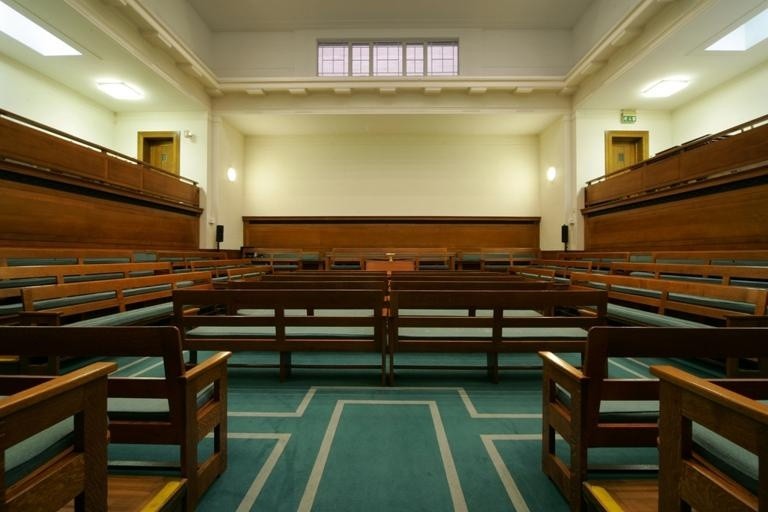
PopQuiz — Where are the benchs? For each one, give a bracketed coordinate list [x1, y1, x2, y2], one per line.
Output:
[0, 362, 184, 512]
[0, 247, 767, 382]
[0, 323, 230, 495]
[536, 325, 767, 511]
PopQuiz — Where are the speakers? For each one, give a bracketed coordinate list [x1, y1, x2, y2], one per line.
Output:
[216, 225, 223, 242]
[562, 226, 568, 242]
